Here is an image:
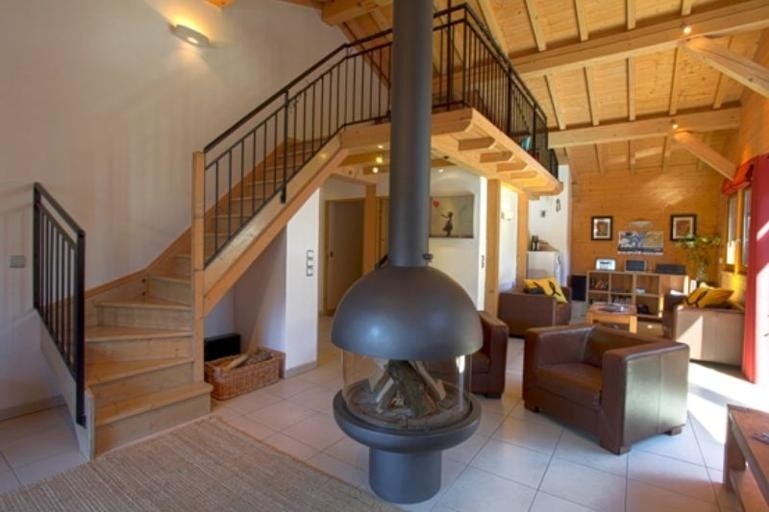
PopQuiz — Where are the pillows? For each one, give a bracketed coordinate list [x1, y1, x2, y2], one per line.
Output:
[684, 282, 713, 304]
[535, 279, 569, 304]
[695, 287, 735, 308]
[523, 277, 549, 294]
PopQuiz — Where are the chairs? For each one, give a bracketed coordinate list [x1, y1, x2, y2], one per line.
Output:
[522, 321, 689, 456]
[427, 311, 509, 398]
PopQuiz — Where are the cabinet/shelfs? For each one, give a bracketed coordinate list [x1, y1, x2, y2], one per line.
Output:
[585, 272, 688, 322]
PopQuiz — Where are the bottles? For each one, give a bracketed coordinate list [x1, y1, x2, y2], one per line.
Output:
[531, 235, 540, 251]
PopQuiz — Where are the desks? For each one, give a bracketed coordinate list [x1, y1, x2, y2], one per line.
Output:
[587, 301, 637, 335]
[724, 404, 769, 512]
[526, 251, 562, 286]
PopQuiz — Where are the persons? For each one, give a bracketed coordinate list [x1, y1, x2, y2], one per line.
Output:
[442, 212, 453, 236]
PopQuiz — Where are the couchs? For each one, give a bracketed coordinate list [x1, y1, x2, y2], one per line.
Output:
[499, 283, 573, 337]
[663, 289, 745, 367]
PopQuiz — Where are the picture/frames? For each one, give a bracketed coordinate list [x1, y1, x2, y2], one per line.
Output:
[670, 215, 697, 242]
[591, 216, 613, 242]
